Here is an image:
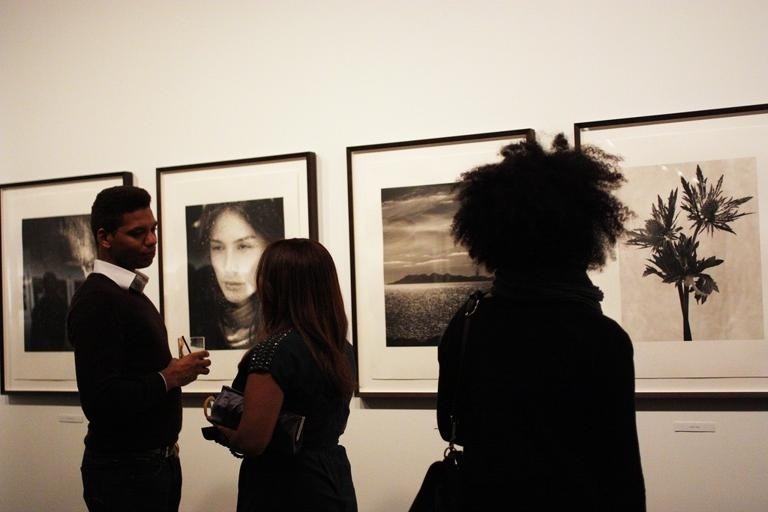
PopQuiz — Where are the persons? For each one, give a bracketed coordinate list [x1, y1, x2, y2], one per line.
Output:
[437, 134, 645, 512]
[67, 187, 210, 512]
[199, 201, 283, 351]
[214, 238, 357, 512]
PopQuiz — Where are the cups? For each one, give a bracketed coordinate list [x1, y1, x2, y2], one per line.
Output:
[178, 337, 206, 358]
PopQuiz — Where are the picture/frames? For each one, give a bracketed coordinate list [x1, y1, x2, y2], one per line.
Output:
[341, 125, 540, 398]
[151, 149, 324, 409]
[1, 167, 139, 401]
[569, 101, 767, 411]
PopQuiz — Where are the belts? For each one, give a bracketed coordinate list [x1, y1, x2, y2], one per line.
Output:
[159, 446, 174, 459]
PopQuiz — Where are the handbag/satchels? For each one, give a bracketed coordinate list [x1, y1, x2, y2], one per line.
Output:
[407, 461, 461, 512]
[202, 386, 305, 467]
